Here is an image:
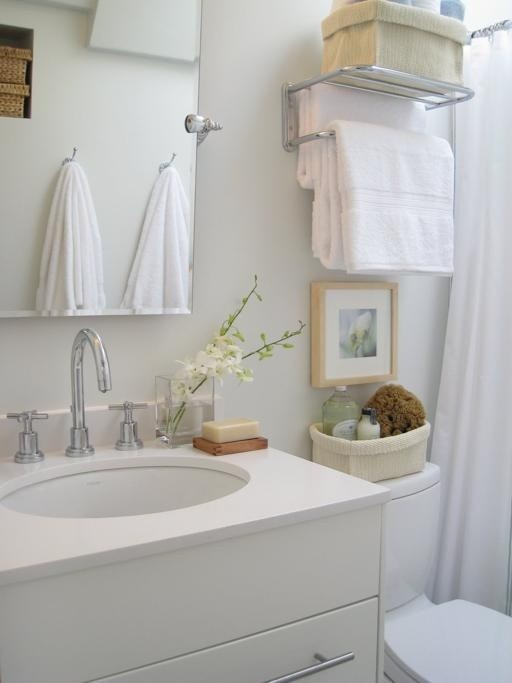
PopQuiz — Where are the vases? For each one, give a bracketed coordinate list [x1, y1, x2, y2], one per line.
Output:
[153, 373, 215, 450]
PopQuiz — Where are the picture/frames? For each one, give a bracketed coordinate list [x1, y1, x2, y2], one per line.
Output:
[309, 278, 398, 388]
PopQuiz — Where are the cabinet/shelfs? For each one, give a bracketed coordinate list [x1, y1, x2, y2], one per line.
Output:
[1, 504, 385, 682]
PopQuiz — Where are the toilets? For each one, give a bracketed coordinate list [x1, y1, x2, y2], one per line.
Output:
[372, 460, 512, 683]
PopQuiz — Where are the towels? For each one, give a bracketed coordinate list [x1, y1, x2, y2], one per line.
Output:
[294, 80, 454, 273]
[120, 168, 191, 315]
[35, 161, 105, 314]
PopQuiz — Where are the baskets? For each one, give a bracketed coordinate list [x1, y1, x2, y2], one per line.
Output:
[309, 419, 431, 483]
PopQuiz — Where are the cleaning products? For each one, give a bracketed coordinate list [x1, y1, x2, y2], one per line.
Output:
[321, 385, 362, 441]
[357, 407, 381, 441]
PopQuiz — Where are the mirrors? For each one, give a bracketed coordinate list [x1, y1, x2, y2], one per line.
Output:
[0, 0, 197, 319]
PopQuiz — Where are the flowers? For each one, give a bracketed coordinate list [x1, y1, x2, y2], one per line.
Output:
[164, 263, 302, 433]
[347, 312, 372, 358]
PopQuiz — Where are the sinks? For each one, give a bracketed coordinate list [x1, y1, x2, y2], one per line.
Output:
[0, 454, 251, 519]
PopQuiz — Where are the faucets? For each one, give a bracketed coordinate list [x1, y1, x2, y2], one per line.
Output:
[65, 327, 112, 458]
